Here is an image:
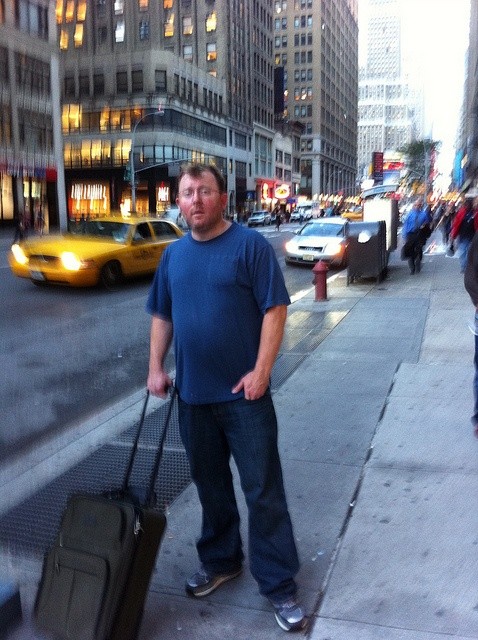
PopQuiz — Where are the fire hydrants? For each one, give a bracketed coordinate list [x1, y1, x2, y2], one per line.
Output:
[312, 258, 330, 301]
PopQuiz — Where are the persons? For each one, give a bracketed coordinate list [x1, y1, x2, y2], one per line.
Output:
[401, 197, 431, 273]
[238, 208, 244, 226]
[273, 205, 282, 229]
[441, 204, 453, 257]
[425, 203, 432, 231]
[144, 162, 309, 631]
[432, 200, 445, 230]
[452, 200, 477, 270]
[299, 206, 304, 224]
[463, 229, 477, 433]
[320, 202, 362, 216]
[14, 214, 25, 245]
[38, 210, 45, 236]
[70, 211, 112, 228]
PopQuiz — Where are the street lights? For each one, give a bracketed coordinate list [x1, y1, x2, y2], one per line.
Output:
[131, 110, 165, 217]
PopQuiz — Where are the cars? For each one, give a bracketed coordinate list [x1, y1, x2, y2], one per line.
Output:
[290, 201, 319, 221]
[284, 218, 349, 268]
[8, 216, 184, 289]
[161, 208, 184, 227]
[248, 210, 273, 225]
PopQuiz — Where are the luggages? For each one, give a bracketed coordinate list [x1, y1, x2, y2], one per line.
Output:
[29, 384, 176, 640]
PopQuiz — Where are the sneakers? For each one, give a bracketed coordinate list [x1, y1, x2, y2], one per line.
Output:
[184, 564, 244, 597]
[266, 593, 306, 631]
